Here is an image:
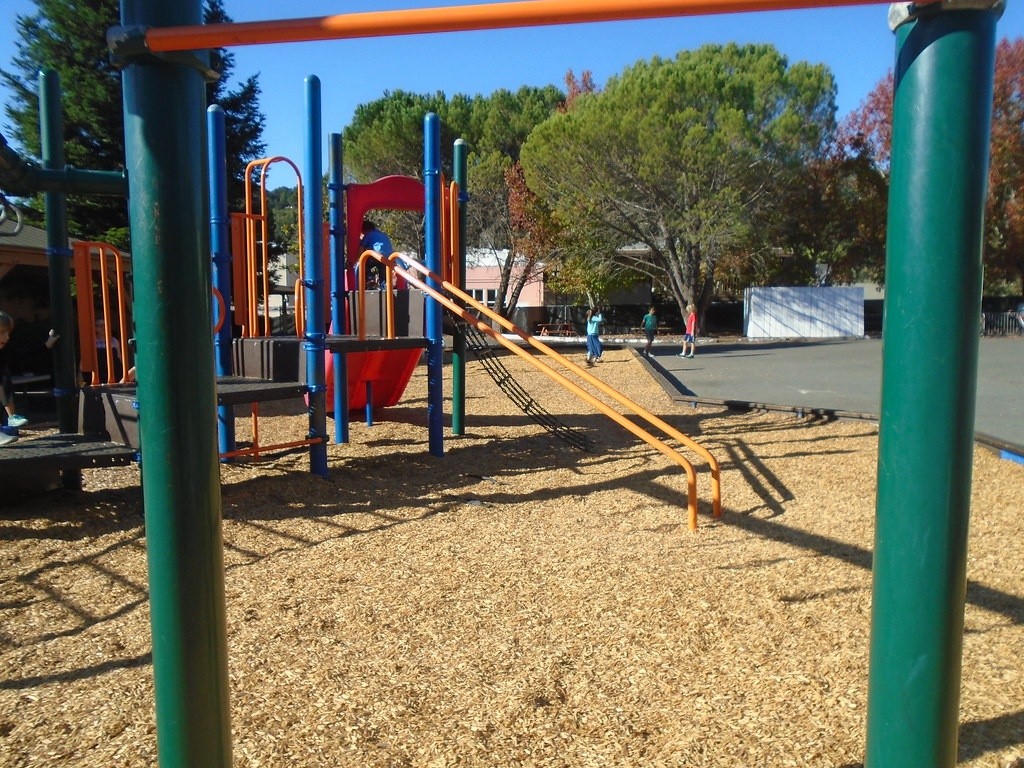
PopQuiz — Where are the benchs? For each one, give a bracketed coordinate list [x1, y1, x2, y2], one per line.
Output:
[536, 330, 542, 332]
[543, 329, 578, 336]
[631, 328, 673, 335]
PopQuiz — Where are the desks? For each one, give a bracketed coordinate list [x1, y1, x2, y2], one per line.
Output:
[537, 322, 574, 336]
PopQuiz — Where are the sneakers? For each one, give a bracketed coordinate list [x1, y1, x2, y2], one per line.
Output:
[675, 354, 686, 358]
[0, 431, 18, 446]
[643, 352, 649, 356]
[648, 353, 654, 357]
[7, 414, 29, 428]
[685, 354, 694, 359]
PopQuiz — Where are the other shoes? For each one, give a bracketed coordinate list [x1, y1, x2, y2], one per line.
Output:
[380, 279, 387, 290]
[586, 359, 594, 364]
[597, 359, 604, 363]
[366, 280, 377, 290]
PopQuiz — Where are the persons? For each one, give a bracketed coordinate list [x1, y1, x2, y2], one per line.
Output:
[583, 306, 604, 367]
[640, 306, 660, 358]
[675, 303, 699, 359]
[359, 220, 395, 289]
[0, 306, 123, 447]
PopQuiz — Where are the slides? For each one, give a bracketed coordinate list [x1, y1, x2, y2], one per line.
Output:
[305, 321, 423, 411]
